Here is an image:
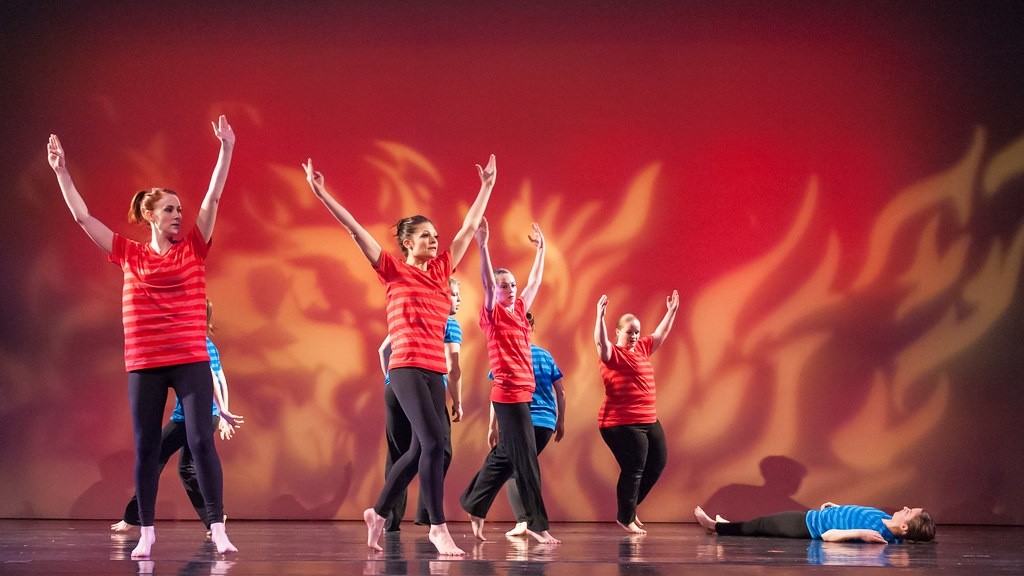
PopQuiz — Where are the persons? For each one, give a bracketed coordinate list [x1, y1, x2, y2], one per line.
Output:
[696, 501, 936, 545]
[593, 287, 681, 534]
[458, 216, 563, 545]
[299, 153, 496, 556]
[47, 114, 239, 557]
[375, 276, 463, 533]
[487, 311, 566, 535]
[111, 295, 244, 540]
